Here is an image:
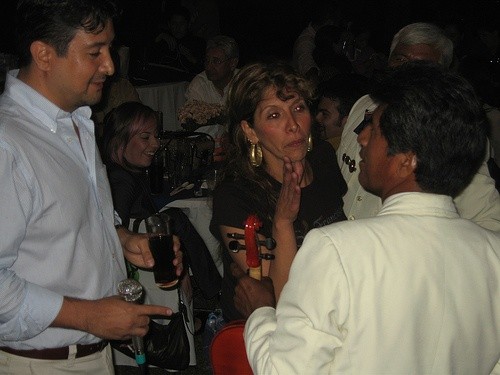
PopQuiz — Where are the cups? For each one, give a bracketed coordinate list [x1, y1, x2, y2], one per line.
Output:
[146, 212, 179, 288]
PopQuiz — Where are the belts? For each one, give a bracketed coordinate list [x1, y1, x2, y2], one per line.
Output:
[0, 340, 109, 360]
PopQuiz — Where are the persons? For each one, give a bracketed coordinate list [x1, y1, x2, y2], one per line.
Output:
[0, 0, 500, 375]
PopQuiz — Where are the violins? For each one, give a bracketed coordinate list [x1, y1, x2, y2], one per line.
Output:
[211, 214, 278, 375]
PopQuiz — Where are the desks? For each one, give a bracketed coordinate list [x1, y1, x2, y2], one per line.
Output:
[136, 77, 189, 133]
